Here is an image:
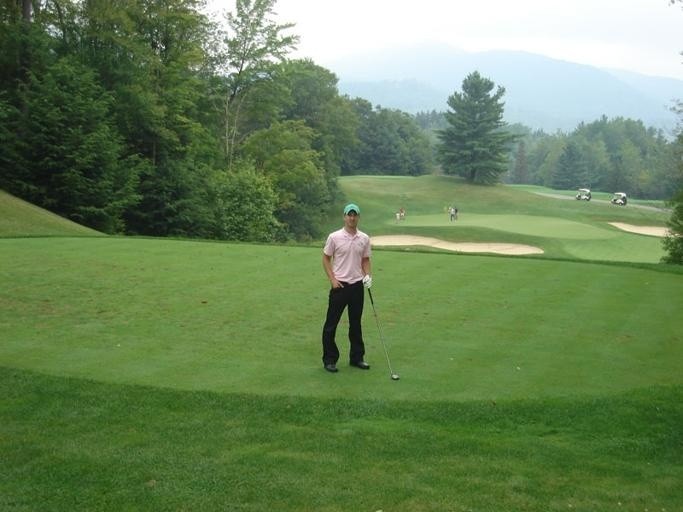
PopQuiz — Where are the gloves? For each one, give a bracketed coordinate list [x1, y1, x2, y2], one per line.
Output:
[362, 274, 372, 289]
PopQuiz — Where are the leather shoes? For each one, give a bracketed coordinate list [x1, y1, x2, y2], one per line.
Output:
[350, 356, 371, 369]
[324, 364, 339, 371]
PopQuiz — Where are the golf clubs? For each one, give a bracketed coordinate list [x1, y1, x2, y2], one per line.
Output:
[368, 288, 400, 379]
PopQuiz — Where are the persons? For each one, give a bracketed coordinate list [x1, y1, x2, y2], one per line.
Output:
[447, 205, 458, 223]
[395, 208, 406, 223]
[322, 201, 374, 373]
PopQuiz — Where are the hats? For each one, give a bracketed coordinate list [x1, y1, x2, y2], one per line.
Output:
[343, 203, 360, 216]
